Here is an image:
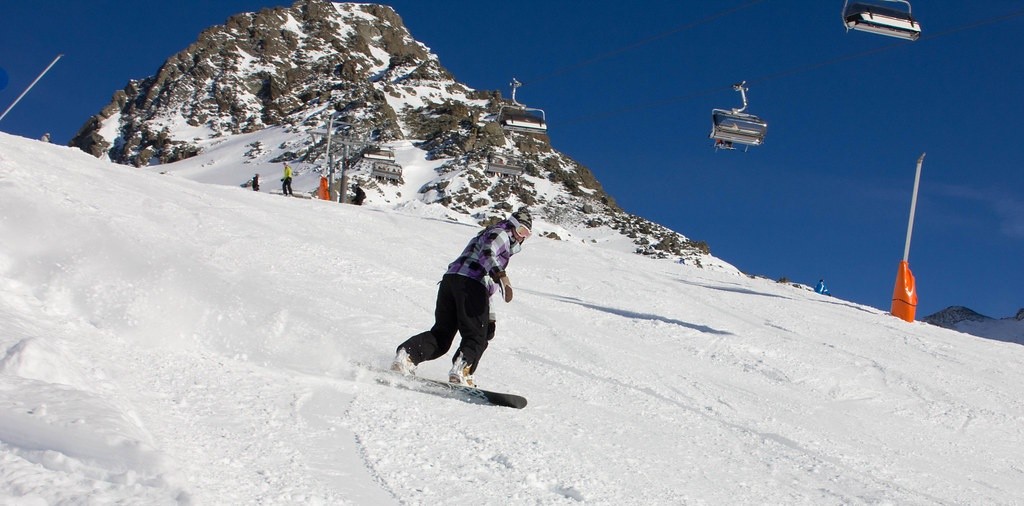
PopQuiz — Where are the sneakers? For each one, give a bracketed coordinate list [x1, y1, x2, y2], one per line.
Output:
[449, 352, 477, 387]
[392, 346, 416, 376]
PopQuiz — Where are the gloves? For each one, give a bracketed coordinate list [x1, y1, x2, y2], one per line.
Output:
[487, 312, 497, 340]
[288, 177, 291, 182]
[281, 178, 284, 181]
[494, 270, 513, 303]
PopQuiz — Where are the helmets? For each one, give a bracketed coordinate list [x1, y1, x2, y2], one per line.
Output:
[283, 161, 288, 167]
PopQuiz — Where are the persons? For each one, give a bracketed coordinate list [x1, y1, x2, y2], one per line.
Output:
[318, 176, 330, 200]
[391, 207, 532, 387]
[814, 279, 831, 297]
[42, 132, 52, 143]
[351, 184, 365, 205]
[281, 163, 293, 197]
[251, 174, 260, 191]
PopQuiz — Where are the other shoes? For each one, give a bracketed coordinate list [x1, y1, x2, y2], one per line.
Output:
[288, 193, 293, 197]
[284, 194, 288, 196]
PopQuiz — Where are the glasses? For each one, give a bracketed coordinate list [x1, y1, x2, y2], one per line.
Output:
[516, 224, 532, 241]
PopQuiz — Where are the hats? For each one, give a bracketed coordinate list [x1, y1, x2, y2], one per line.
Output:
[508, 206, 533, 229]
[255, 174, 260, 177]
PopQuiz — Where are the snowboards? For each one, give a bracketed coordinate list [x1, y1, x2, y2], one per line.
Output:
[347, 359, 528, 409]
